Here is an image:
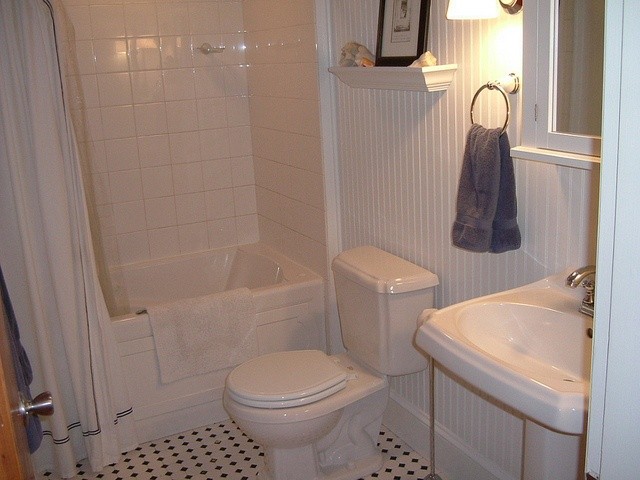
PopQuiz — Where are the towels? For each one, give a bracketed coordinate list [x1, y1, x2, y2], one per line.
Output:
[451, 123, 522, 253]
[136, 286, 259, 385]
[0, 270, 43, 455]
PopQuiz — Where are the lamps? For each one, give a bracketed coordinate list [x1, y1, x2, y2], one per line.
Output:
[446, 0, 523, 22]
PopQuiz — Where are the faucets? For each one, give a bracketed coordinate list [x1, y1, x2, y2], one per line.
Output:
[566, 265, 596, 318]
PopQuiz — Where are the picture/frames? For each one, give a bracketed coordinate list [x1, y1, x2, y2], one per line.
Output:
[375, 0, 430, 66]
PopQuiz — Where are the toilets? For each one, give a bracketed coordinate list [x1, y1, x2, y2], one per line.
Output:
[221, 243, 439, 479]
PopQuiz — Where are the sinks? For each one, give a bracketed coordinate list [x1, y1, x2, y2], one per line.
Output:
[416, 271, 596, 437]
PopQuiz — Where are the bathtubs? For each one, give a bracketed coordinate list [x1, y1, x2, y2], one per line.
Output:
[99, 243, 328, 444]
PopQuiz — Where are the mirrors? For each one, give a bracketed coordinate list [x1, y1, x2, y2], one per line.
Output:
[523, 1, 604, 158]
